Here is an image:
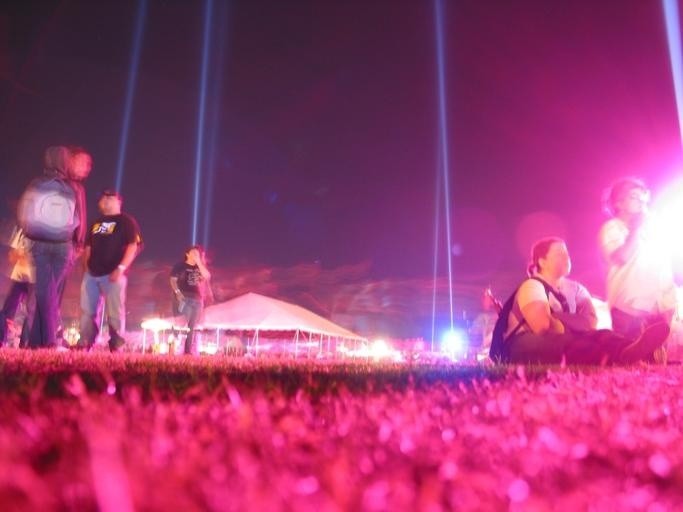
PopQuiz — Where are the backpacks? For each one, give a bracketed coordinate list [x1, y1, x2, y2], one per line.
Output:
[24, 176, 81, 243]
[489, 276, 549, 365]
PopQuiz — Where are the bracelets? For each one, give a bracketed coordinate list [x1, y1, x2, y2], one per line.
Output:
[118, 264, 126, 271]
[174, 289, 180, 293]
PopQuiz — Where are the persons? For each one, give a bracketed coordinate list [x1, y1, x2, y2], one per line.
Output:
[599, 177, 683, 363]
[75, 191, 144, 350]
[0, 145, 93, 350]
[489, 237, 670, 364]
[170, 246, 211, 356]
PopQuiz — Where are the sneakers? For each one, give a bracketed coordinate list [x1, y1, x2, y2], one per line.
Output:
[620, 322, 670, 365]
